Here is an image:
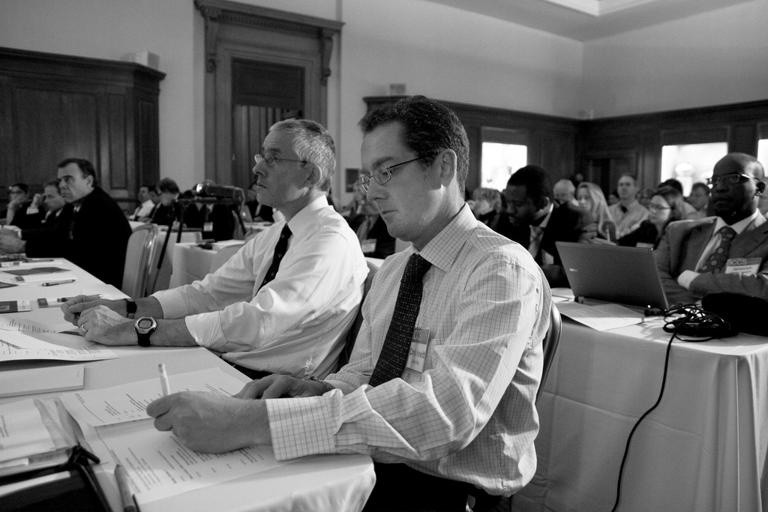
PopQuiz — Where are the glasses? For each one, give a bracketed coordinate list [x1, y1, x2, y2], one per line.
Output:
[703, 171, 750, 186]
[254, 152, 306, 167]
[358, 155, 422, 190]
[646, 203, 669, 210]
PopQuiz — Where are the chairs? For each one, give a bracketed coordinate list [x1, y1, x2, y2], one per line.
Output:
[348, 261, 379, 358]
[538, 304, 564, 397]
[121, 223, 158, 297]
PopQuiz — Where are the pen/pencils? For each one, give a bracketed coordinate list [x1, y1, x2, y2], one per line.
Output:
[115, 464, 140, 512]
[41, 279, 76, 286]
[157, 363, 169, 397]
[56, 297, 71, 303]
[31, 259, 53, 261]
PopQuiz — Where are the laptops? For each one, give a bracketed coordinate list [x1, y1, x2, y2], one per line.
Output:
[554, 241, 688, 316]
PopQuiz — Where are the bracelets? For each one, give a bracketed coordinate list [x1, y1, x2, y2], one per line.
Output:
[121, 297, 139, 319]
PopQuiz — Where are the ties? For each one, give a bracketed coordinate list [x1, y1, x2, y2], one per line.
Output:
[368, 253, 432, 387]
[697, 227, 736, 273]
[529, 227, 542, 257]
[358, 217, 371, 242]
[255, 224, 292, 295]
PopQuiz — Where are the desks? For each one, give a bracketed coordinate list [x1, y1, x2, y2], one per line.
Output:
[0, 256, 377, 511]
[169, 240, 244, 290]
[129, 219, 203, 290]
[510, 286, 768, 512]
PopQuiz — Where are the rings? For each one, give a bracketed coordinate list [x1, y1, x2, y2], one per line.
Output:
[80, 323, 88, 333]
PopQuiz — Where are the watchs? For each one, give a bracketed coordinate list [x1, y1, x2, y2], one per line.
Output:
[133, 315, 158, 345]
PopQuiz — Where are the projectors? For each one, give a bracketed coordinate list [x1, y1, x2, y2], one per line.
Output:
[189, 183, 245, 203]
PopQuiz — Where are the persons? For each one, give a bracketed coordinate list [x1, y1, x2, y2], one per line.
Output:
[60, 118, 367, 382]
[654, 152, 767, 306]
[1, 158, 768, 290]
[146, 94, 551, 511]
